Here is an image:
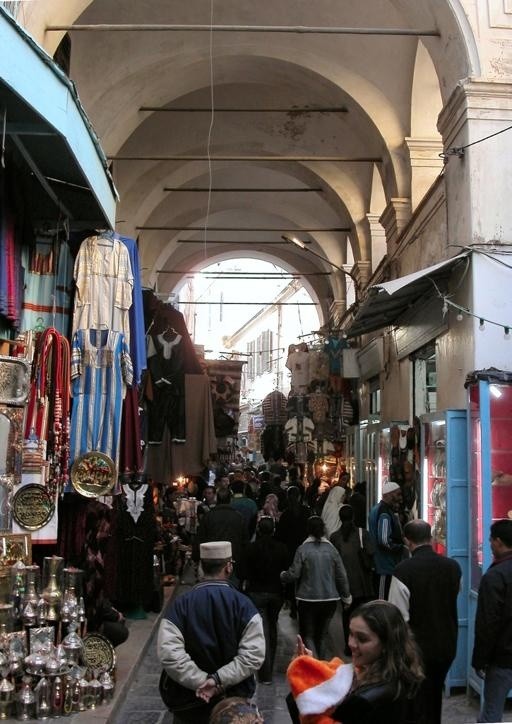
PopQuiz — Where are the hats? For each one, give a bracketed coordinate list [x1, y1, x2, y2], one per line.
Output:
[381, 479, 400, 495]
[198, 539, 235, 562]
[287, 655, 356, 718]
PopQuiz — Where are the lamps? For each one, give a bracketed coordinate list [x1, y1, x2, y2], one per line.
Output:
[280, 231, 351, 274]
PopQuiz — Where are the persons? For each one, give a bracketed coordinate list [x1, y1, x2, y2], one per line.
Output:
[158, 442, 512, 723]
